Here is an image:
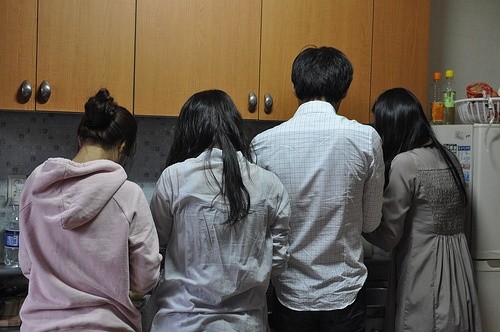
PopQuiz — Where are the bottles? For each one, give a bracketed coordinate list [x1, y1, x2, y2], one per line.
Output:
[431, 71, 444, 125]
[442, 69, 456, 125]
[4, 183, 23, 270]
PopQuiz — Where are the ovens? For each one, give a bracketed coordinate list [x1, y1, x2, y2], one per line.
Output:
[262, 279, 397, 332]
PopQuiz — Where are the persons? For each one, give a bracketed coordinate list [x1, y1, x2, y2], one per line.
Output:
[18, 87, 163, 332]
[362, 87, 483, 332]
[249, 44, 385, 332]
[150, 89, 292, 332]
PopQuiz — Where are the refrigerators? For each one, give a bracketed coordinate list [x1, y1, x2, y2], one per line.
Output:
[428, 122, 500, 332]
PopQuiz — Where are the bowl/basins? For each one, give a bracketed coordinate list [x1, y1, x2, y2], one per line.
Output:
[465, 82, 493, 99]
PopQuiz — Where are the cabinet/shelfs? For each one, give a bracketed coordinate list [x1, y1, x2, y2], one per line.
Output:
[0, 0, 431, 125]
